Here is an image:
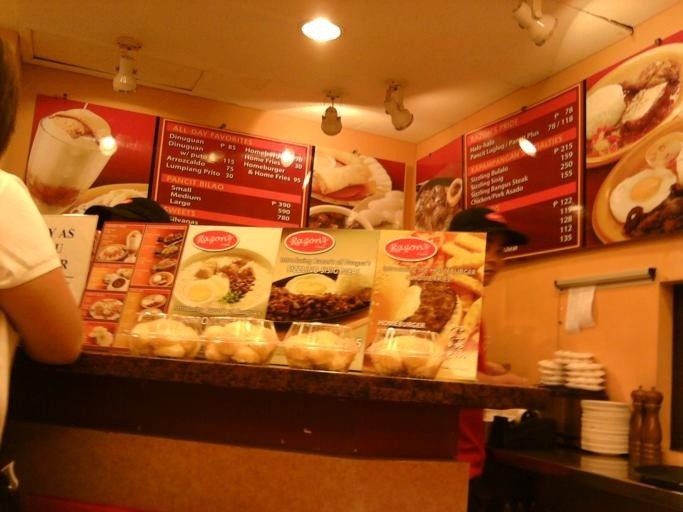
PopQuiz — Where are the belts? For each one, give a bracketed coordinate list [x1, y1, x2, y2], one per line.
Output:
[124, 236, 142, 263]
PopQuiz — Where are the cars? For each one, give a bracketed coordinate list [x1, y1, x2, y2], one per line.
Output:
[23, 115, 118, 214]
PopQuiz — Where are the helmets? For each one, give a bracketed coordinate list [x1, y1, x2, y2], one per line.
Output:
[308, 157, 404, 231]
[587, 44, 683, 246]
[579, 398, 632, 456]
[90, 245, 272, 319]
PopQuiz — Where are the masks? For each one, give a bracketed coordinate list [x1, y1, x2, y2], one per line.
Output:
[431, 233, 486, 352]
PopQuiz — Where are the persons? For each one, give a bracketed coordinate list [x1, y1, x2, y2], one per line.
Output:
[0, 36, 83, 449]
[84, 194, 171, 231]
[451, 207, 532, 512]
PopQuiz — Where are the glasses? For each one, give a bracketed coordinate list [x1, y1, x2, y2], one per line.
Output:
[110, 37, 144, 92]
[511, 0, 558, 48]
[317, 83, 414, 136]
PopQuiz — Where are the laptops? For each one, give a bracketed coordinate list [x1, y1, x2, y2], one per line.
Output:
[584, 84, 625, 140]
[622, 82, 670, 128]
